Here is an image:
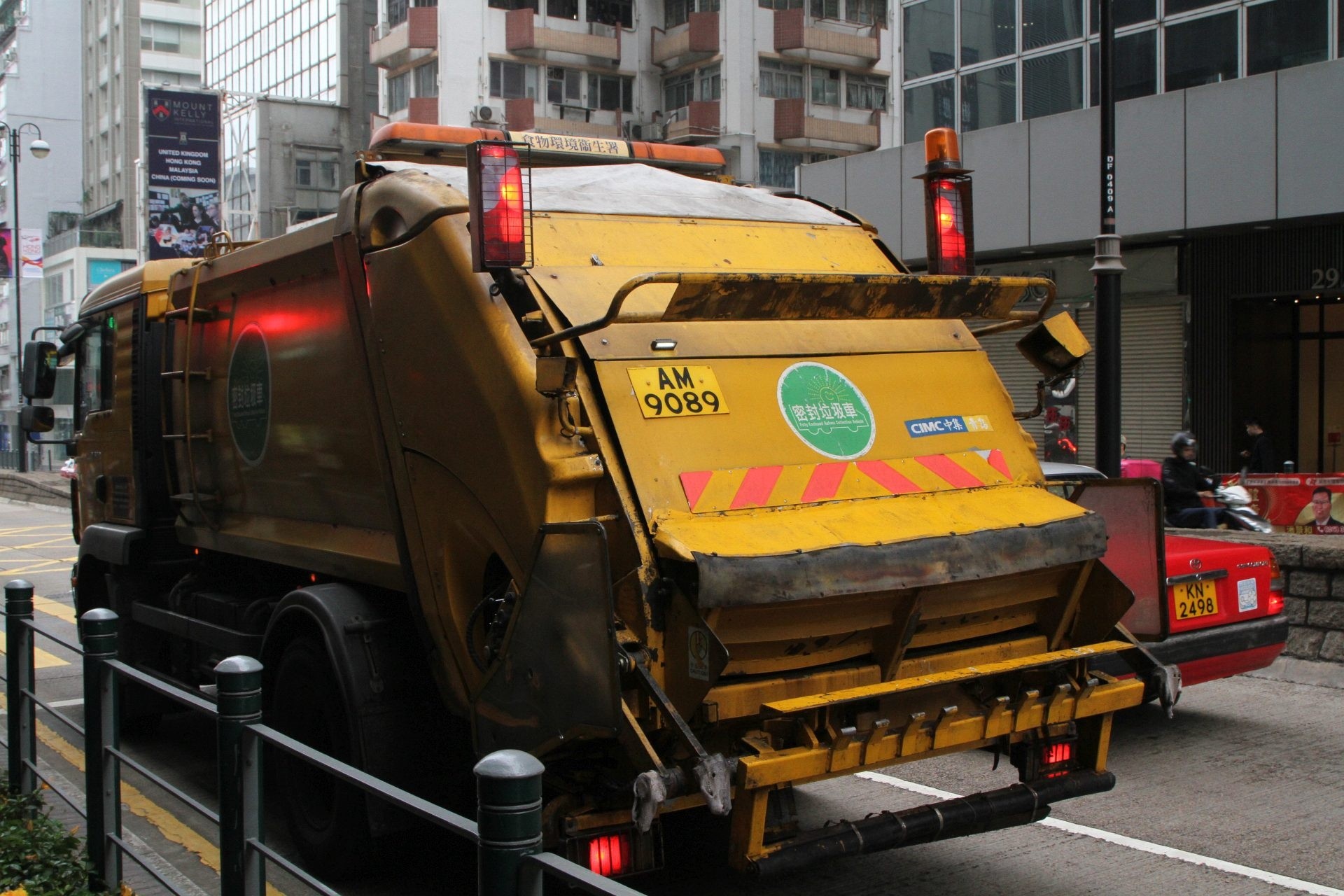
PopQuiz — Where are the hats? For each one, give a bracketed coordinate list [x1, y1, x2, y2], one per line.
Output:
[1121, 434, 1127, 451]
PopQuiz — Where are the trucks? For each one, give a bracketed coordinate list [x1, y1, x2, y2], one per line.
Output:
[17, 122, 1144, 896]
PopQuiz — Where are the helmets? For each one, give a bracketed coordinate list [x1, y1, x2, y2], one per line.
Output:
[1171, 432, 1197, 454]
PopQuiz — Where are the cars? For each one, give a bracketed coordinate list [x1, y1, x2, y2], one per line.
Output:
[1039, 458, 1292, 712]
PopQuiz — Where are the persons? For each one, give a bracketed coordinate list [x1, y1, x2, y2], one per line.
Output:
[1303, 486, 1344, 526]
[1161, 431, 1243, 529]
[149, 192, 219, 231]
[1241, 419, 1279, 473]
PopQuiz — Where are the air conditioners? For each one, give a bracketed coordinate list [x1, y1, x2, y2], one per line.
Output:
[588, 22, 611, 37]
[640, 124, 665, 142]
[472, 104, 503, 126]
[625, 119, 641, 142]
